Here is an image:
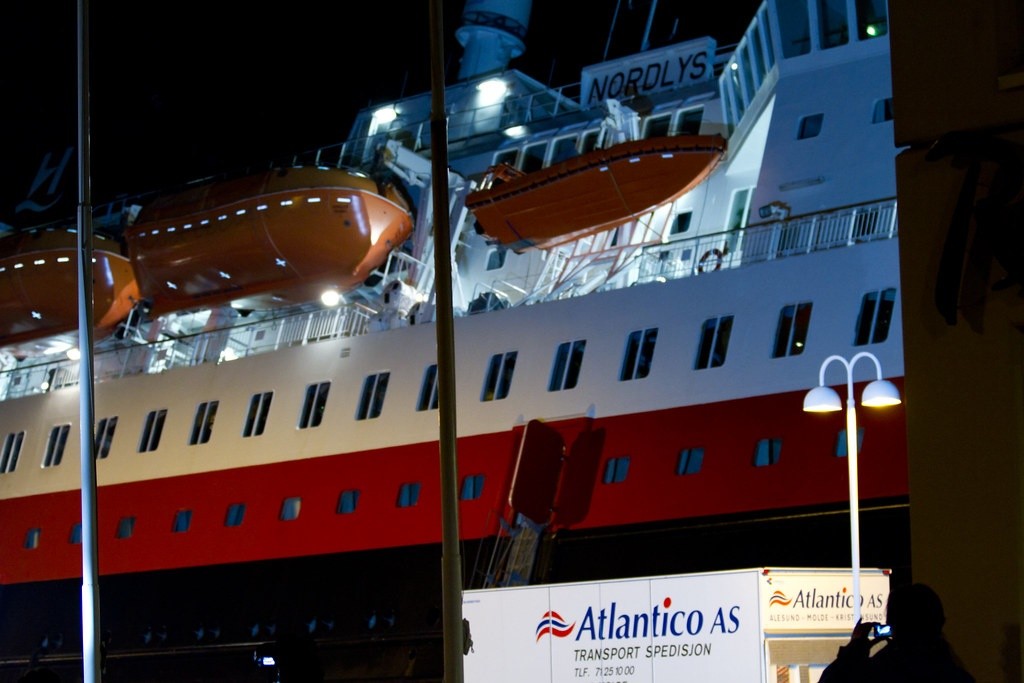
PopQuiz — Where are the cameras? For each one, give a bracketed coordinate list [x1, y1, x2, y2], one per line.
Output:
[255, 652, 275, 667]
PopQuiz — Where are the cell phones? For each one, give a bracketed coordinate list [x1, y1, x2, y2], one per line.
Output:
[874, 624, 892, 638]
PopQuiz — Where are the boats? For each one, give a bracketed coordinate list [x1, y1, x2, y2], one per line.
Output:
[118, 160, 415, 326]
[467, 99, 728, 255]
[0, 224, 139, 352]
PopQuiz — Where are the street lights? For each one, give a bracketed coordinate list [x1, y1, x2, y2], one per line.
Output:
[803, 350, 900, 635]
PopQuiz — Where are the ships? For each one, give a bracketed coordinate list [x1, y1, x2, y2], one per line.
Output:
[0, 0, 914, 662]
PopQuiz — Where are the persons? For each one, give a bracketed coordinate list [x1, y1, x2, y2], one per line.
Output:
[817, 583, 973, 683]
